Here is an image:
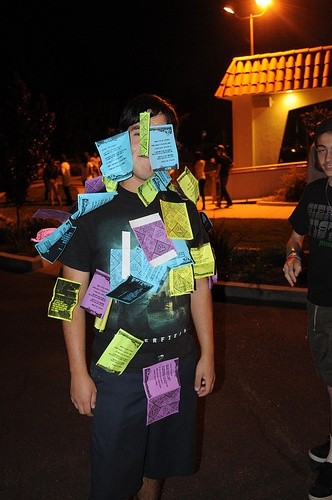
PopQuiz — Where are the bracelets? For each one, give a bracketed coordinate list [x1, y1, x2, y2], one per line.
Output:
[286, 248, 301, 257]
[287, 255, 301, 260]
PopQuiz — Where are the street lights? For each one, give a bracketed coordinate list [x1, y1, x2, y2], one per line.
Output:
[223, 0, 272, 56]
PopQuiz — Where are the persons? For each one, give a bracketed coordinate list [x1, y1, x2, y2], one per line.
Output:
[194, 145, 232, 210]
[283, 120, 332, 500]
[27, 149, 101, 206]
[31, 95, 218, 500]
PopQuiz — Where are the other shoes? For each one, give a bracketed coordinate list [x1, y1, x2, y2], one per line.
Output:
[309, 443, 330, 463]
[308, 476, 332, 500]
[214, 202, 221, 209]
[224, 203, 233, 208]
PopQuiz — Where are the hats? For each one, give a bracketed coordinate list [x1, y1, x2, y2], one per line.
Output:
[215, 145, 224, 151]
